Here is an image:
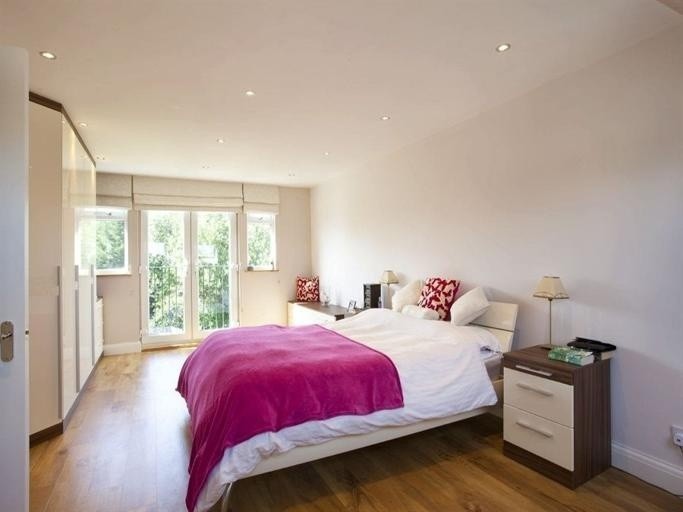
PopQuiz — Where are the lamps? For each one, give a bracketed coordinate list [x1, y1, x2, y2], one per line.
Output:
[531, 274, 570, 348]
[378, 269, 400, 305]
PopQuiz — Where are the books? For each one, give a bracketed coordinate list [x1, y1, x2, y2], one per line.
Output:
[567, 336, 617, 361]
[363, 283, 381, 309]
[548, 346, 595, 366]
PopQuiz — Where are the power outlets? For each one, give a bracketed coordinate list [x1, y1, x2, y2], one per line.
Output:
[668, 422, 681, 446]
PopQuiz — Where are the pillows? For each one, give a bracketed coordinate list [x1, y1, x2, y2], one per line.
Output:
[390, 276, 422, 309]
[449, 287, 490, 326]
[294, 275, 319, 301]
[417, 277, 460, 321]
[401, 303, 438, 324]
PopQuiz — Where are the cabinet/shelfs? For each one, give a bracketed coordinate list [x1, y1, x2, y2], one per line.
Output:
[29, 89, 97, 447]
[286, 298, 357, 326]
[92, 288, 106, 364]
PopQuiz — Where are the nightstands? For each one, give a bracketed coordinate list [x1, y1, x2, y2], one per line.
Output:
[501, 345, 612, 489]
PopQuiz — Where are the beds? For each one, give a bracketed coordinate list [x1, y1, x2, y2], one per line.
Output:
[173, 278, 522, 511]
